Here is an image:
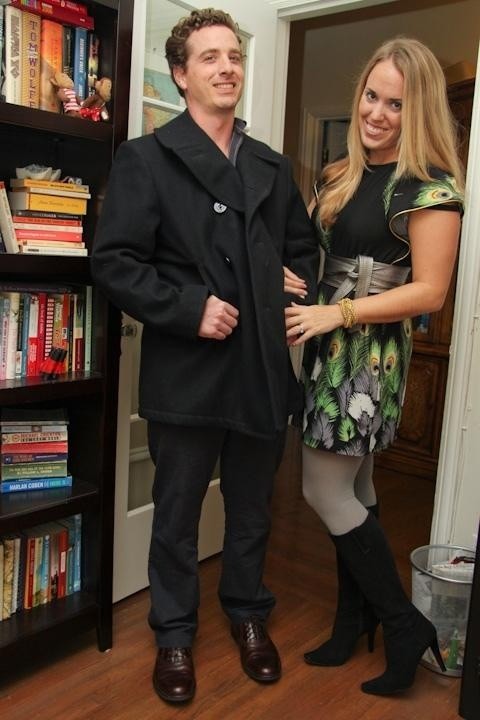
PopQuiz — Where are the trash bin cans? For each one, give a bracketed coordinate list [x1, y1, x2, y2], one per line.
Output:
[410, 544, 475, 678]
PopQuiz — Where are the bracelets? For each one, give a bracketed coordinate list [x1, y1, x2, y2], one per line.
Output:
[336, 298, 358, 329]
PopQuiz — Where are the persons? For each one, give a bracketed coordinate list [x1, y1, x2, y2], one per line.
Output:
[283, 39, 466, 696]
[91, 8, 320, 704]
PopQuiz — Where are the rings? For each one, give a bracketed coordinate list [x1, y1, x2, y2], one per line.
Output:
[300, 324, 304, 334]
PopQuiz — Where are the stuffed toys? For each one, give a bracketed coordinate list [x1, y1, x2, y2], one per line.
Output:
[49, 72, 112, 122]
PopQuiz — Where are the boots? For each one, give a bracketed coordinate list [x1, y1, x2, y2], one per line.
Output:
[304, 504, 447, 694]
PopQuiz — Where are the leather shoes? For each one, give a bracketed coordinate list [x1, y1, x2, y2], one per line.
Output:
[153, 647, 195, 703]
[231, 616, 281, 682]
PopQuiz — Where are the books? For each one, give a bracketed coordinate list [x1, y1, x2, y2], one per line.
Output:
[0, 286, 92, 379]
[0, 179, 91, 257]
[1, 0, 99, 118]
[0, 408, 74, 493]
[0, 513, 82, 622]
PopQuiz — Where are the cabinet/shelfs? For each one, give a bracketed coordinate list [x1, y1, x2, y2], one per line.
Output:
[1, 1, 133, 656]
